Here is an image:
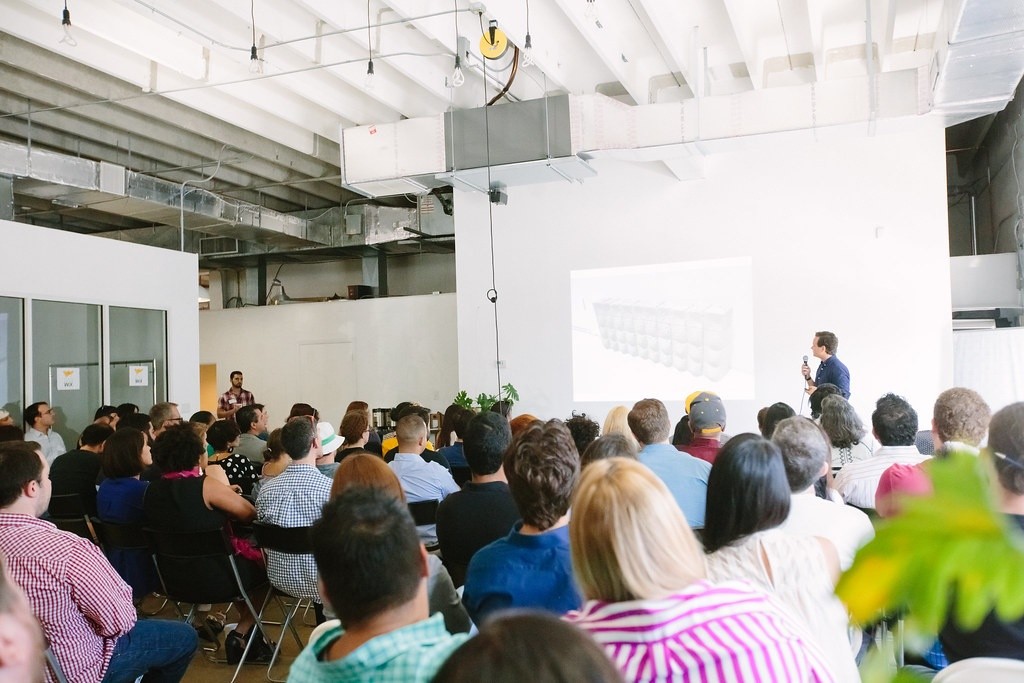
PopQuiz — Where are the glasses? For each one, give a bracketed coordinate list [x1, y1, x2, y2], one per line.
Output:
[167, 417, 184, 424]
[39, 408, 54, 415]
[363, 425, 373, 432]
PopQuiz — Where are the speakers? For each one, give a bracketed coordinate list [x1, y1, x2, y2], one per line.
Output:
[488, 192, 508, 206]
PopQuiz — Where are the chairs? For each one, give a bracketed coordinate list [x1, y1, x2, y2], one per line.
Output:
[408, 499, 441, 552]
[48, 492, 326, 683]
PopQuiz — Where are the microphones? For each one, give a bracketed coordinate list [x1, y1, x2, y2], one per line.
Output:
[803, 355, 809, 375]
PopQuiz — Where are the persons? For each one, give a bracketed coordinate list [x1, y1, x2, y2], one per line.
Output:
[217, 371, 254, 423]
[800, 330, 853, 421]
[0, 382, 1023, 682]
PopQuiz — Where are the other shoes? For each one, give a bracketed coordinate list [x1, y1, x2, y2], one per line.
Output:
[198, 604, 211, 611]
[190, 613, 230, 652]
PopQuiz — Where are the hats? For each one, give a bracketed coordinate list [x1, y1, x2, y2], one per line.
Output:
[683, 392, 729, 434]
[317, 422, 345, 456]
[876, 463, 934, 520]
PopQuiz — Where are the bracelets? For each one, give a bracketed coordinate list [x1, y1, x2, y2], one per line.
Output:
[807, 377, 811, 380]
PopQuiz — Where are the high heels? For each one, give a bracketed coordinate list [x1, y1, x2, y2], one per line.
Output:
[225, 624, 281, 665]
